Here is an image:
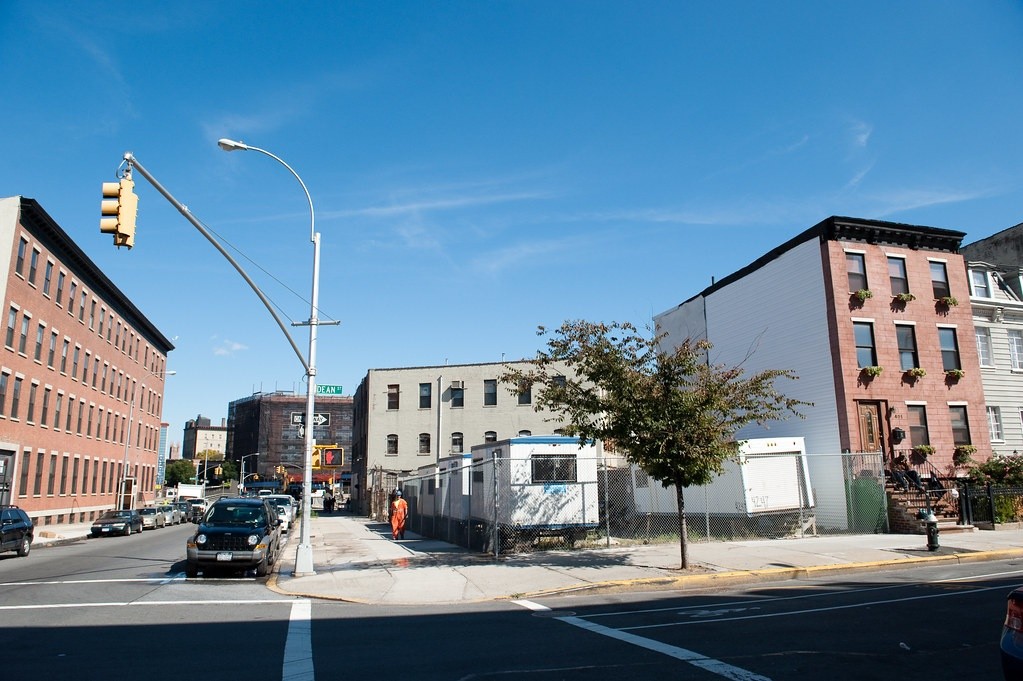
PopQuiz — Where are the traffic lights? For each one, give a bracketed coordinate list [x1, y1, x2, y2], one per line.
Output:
[101, 183, 119, 232]
[280, 466, 284, 473]
[276, 466, 280, 473]
[219, 468, 222, 474]
[324, 448, 344, 466]
[215, 468, 218, 474]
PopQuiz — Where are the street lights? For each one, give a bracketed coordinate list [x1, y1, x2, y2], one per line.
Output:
[243, 473, 257, 489]
[118, 371, 176, 516]
[236, 459, 244, 487]
[240, 453, 260, 492]
[218, 137, 317, 577]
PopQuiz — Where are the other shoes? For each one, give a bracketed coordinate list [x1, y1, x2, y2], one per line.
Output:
[392, 536, 398, 540]
[400, 533, 404, 539]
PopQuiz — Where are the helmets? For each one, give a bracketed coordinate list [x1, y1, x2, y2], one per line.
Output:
[396, 490, 402, 495]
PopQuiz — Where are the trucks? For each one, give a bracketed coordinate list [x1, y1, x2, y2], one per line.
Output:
[177, 481, 205, 520]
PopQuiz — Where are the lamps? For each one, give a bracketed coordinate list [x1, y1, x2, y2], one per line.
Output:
[892, 426, 905, 440]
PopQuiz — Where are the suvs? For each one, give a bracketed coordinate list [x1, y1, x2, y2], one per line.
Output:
[0, 505, 34, 557]
[187, 496, 280, 577]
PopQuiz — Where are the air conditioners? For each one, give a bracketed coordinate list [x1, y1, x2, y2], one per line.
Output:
[450, 380, 462, 388]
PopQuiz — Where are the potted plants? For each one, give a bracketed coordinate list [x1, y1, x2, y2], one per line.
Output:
[945, 368, 964, 383]
[913, 444, 935, 464]
[859, 365, 882, 382]
[906, 367, 926, 382]
[938, 296, 958, 306]
[896, 292, 915, 301]
[954, 444, 977, 466]
[853, 288, 872, 301]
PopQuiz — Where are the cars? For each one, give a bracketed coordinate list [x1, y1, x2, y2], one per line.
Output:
[258, 496, 267, 499]
[999, 587, 1023, 681]
[91, 510, 144, 536]
[168, 502, 193, 523]
[258, 490, 272, 496]
[159, 505, 182, 526]
[263, 495, 298, 550]
[136, 507, 165, 530]
[240, 491, 250, 499]
[185, 498, 209, 516]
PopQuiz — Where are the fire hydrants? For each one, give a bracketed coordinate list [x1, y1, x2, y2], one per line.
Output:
[925, 510, 940, 552]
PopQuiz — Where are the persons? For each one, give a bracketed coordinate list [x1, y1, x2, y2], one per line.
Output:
[390, 492, 408, 540]
[322, 496, 336, 514]
[890, 452, 925, 492]
[346, 498, 350, 509]
